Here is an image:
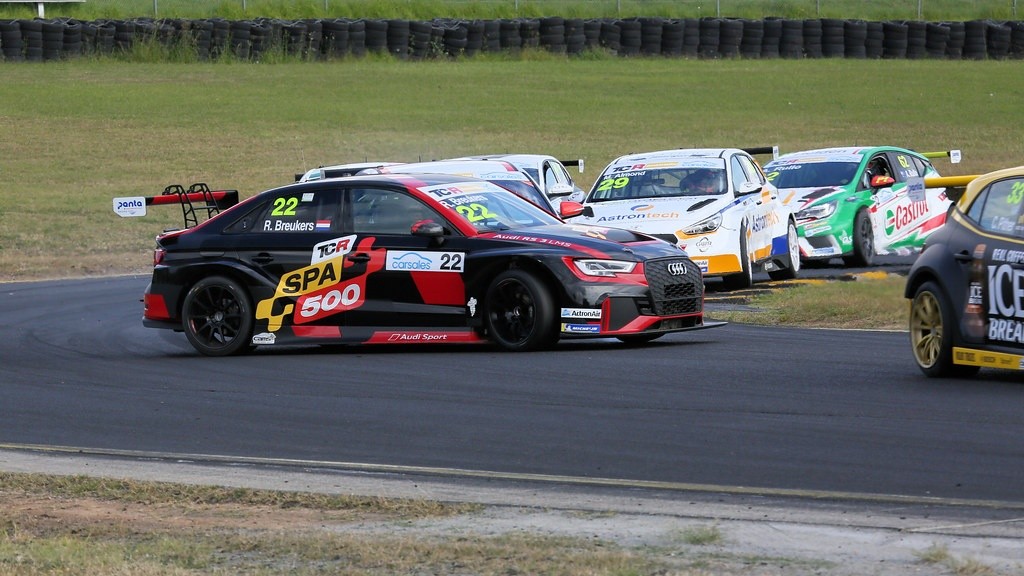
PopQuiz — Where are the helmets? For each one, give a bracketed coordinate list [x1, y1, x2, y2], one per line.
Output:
[855, 161, 876, 180]
[690, 168, 717, 193]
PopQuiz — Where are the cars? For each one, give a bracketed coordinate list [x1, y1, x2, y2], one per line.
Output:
[355, 160, 587, 219]
[113, 175, 728, 356]
[904, 164, 1024, 378]
[759, 146, 961, 267]
[570, 146, 800, 290]
[444, 153, 587, 218]
[294, 163, 407, 185]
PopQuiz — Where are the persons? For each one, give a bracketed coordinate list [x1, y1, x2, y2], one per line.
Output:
[688, 169, 715, 193]
